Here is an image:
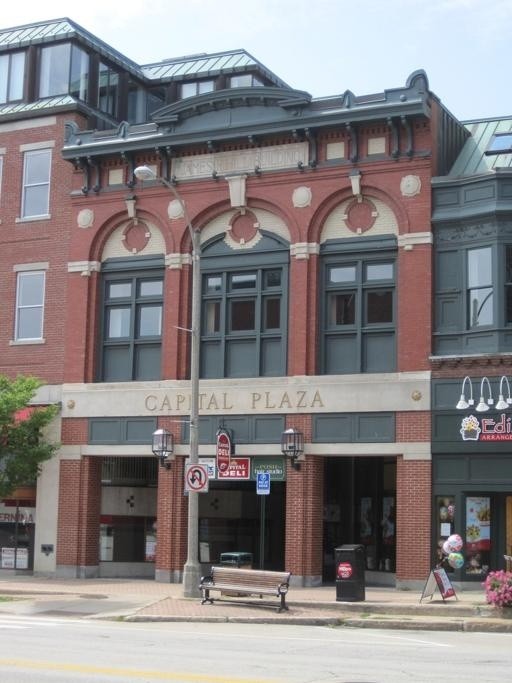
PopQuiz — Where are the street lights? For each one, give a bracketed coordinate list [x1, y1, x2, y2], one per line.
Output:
[133, 165, 206, 599]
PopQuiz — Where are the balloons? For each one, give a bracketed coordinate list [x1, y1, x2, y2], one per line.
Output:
[443, 534, 464, 569]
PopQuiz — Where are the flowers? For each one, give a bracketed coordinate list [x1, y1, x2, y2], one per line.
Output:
[482, 569, 512, 609]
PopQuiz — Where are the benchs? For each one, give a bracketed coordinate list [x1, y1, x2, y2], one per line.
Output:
[199, 566, 293, 613]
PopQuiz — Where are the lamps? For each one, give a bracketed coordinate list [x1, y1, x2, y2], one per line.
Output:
[152, 426, 174, 469]
[281, 425, 306, 471]
[455, 376, 512, 412]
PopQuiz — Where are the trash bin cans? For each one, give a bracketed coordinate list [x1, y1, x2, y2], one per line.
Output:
[219, 551, 254, 597]
[335, 544, 367, 601]
